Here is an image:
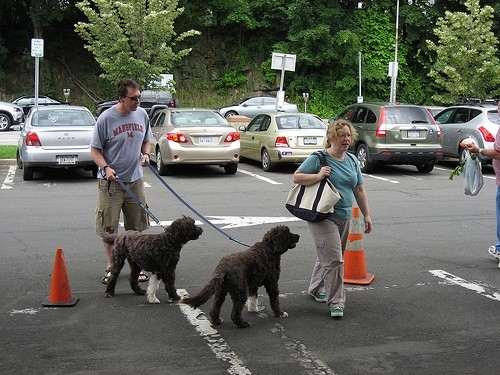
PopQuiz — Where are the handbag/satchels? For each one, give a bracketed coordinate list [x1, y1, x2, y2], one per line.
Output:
[285, 150, 341, 222]
[463, 149, 483, 196]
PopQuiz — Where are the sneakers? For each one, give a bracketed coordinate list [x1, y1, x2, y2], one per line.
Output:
[331, 306, 343, 316]
[488, 245, 500, 258]
[309, 291, 326, 301]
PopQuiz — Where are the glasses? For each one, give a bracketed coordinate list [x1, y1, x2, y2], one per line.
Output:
[125, 94, 142, 100]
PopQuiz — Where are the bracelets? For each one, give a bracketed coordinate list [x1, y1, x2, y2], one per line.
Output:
[143, 153, 151, 159]
[479, 148, 484, 155]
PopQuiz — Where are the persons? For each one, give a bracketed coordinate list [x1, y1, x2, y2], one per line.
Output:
[466, 100, 500, 269]
[90, 79, 153, 283]
[293, 119, 372, 317]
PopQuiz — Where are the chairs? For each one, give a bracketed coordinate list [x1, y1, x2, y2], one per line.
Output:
[40, 119, 85, 125]
[175, 117, 219, 124]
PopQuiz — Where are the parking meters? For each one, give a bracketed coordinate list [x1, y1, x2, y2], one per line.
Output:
[63, 88, 71, 105]
[303, 93, 309, 112]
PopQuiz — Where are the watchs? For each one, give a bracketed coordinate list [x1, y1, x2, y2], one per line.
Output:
[102, 165, 109, 172]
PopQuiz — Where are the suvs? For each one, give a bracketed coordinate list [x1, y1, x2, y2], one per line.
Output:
[93, 89, 177, 119]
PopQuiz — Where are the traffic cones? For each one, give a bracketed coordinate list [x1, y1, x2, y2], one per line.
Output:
[39, 247, 80, 307]
[340, 205, 375, 285]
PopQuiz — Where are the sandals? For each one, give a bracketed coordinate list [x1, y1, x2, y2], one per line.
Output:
[138, 269, 149, 281]
[102, 267, 111, 284]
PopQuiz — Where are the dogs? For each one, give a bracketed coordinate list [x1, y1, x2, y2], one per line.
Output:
[179, 222, 300, 328]
[94, 210, 203, 305]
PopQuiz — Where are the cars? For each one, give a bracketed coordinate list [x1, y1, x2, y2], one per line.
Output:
[237, 113, 331, 171]
[147, 109, 241, 175]
[328, 98, 500, 174]
[220, 97, 300, 120]
[0, 96, 101, 181]
